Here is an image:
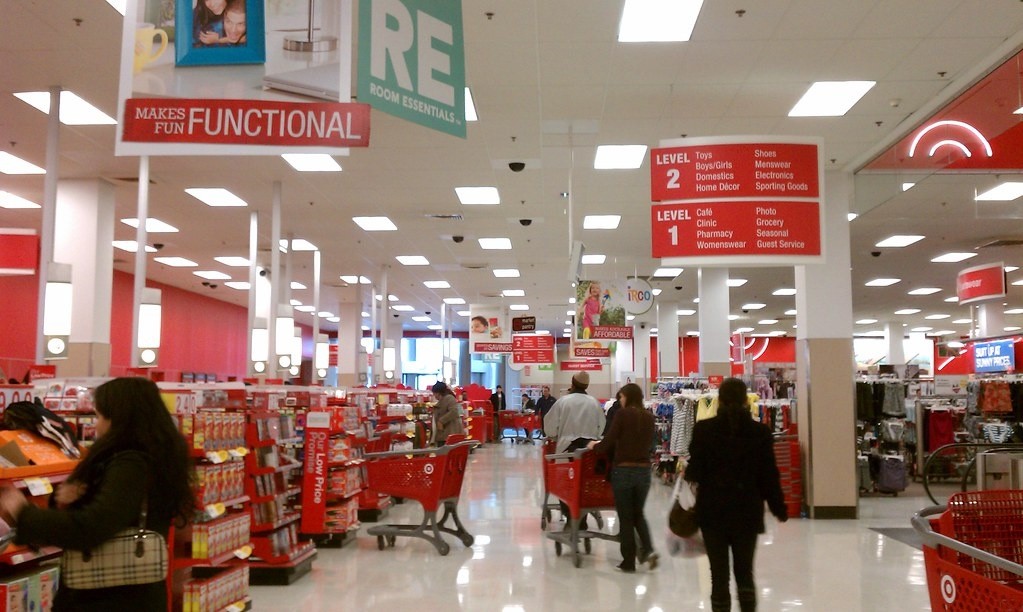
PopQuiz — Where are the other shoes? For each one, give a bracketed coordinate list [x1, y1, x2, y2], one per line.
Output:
[648, 555, 659, 569]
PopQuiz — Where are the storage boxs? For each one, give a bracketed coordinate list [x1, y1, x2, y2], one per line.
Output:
[324, 497, 359, 531]
[182, 410, 244, 450]
[191, 511, 250, 559]
[772, 433, 802, 518]
[188, 461, 245, 507]
[181, 565, 250, 612]
[328, 465, 367, 494]
[324, 405, 361, 432]
[255, 416, 298, 556]
[325, 434, 362, 462]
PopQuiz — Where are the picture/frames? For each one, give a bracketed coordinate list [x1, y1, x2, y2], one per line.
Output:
[173, 0, 267, 68]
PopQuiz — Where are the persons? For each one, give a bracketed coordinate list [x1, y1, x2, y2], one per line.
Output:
[914, 389, 921, 399]
[493, 384, 557, 444]
[429, 381, 464, 447]
[588, 384, 659, 572]
[684, 377, 788, 611]
[472, 316, 488, 333]
[193, 0, 246, 47]
[0, 377, 195, 612]
[544, 373, 605, 530]
[578, 284, 604, 326]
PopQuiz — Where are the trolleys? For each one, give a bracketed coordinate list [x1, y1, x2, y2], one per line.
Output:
[909, 488, 1023, 612]
[497, 409, 542, 446]
[543, 446, 641, 567]
[539, 438, 562, 531]
[363, 433, 482, 556]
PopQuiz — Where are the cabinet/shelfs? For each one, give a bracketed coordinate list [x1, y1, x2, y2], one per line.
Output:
[340, 390, 397, 523]
[0, 415, 90, 612]
[0, 385, 252, 612]
[243, 384, 319, 586]
[512, 387, 543, 412]
[300, 388, 362, 550]
[398, 392, 473, 455]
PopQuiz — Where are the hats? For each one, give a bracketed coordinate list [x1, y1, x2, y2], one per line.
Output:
[572, 370, 591, 387]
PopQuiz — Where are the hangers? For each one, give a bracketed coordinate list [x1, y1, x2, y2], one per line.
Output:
[857, 373, 901, 384]
[886, 451, 904, 462]
[924, 403, 950, 413]
[969, 373, 1023, 383]
[857, 449, 868, 461]
[758, 398, 790, 408]
[670, 387, 720, 400]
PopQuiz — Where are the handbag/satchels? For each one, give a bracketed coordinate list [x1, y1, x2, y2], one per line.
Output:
[668, 473, 700, 537]
[58, 450, 166, 590]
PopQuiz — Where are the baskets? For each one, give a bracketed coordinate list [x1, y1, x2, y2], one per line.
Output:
[764, 435, 805, 517]
